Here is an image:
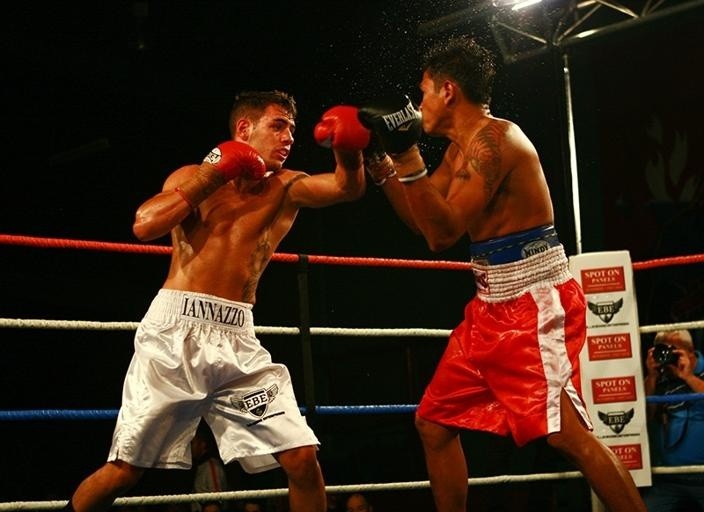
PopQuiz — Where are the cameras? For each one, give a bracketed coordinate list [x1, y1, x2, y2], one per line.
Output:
[653, 341, 679, 382]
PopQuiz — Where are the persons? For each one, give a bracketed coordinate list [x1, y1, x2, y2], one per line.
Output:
[357, 39, 647, 512]
[644, 329, 704, 511]
[62, 91, 371, 512]
[165, 430, 374, 512]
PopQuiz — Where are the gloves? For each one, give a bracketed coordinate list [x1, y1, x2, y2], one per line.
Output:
[173, 138, 268, 210]
[356, 91, 434, 184]
[317, 104, 371, 171]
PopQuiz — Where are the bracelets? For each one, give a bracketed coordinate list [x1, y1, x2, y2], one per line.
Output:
[399, 168, 427, 182]
[375, 170, 397, 185]
